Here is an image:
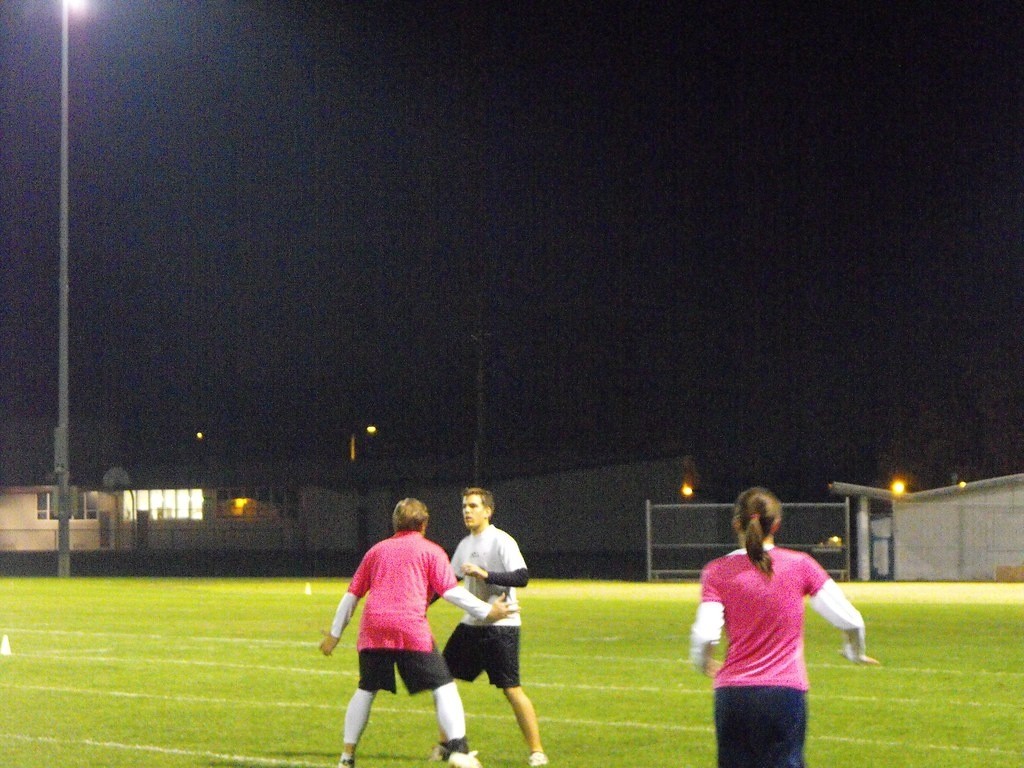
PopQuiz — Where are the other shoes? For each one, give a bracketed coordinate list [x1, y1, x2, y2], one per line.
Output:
[449, 750, 482, 768]
[338, 756, 354, 768]
[528, 752, 548, 767]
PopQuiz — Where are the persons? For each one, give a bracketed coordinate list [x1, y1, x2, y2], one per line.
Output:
[430, 488, 550, 766]
[319, 498, 522, 767]
[690, 487, 880, 768]
